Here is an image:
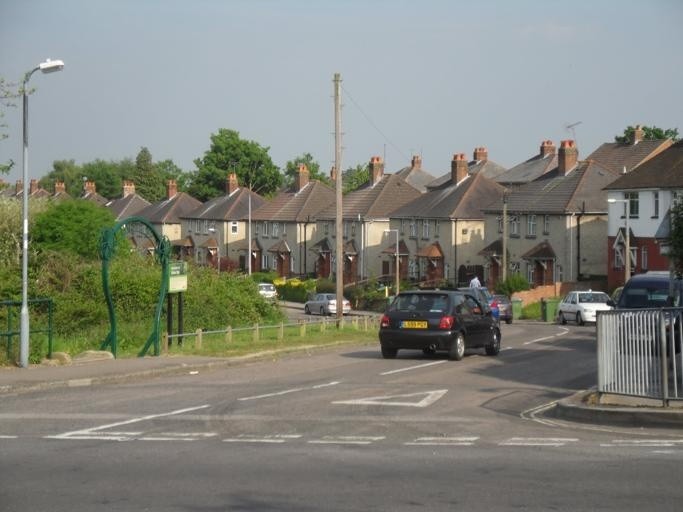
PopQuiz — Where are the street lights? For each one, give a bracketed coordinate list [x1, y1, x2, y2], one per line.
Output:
[18, 57, 66, 367]
[606, 197, 630, 284]
[383, 228, 399, 295]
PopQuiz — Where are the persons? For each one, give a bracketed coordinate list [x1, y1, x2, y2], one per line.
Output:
[469, 276, 482, 287]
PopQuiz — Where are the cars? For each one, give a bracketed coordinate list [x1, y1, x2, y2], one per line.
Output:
[256, 282, 278, 306]
[556, 289, 613, 327]
[455, 287, 493, 320]
[479, 287, 502, 329]
[303, 292, 352, 316]
[493, 294, 513, 323]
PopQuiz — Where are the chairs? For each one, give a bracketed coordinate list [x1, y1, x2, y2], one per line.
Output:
[415, 300, 434, 311]
[626, 294, 649, 304]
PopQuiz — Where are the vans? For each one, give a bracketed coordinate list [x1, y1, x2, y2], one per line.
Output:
[610, 274, 681, 355]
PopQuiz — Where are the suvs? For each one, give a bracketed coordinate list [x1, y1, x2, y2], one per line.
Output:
[378, 286, 500, 361]
